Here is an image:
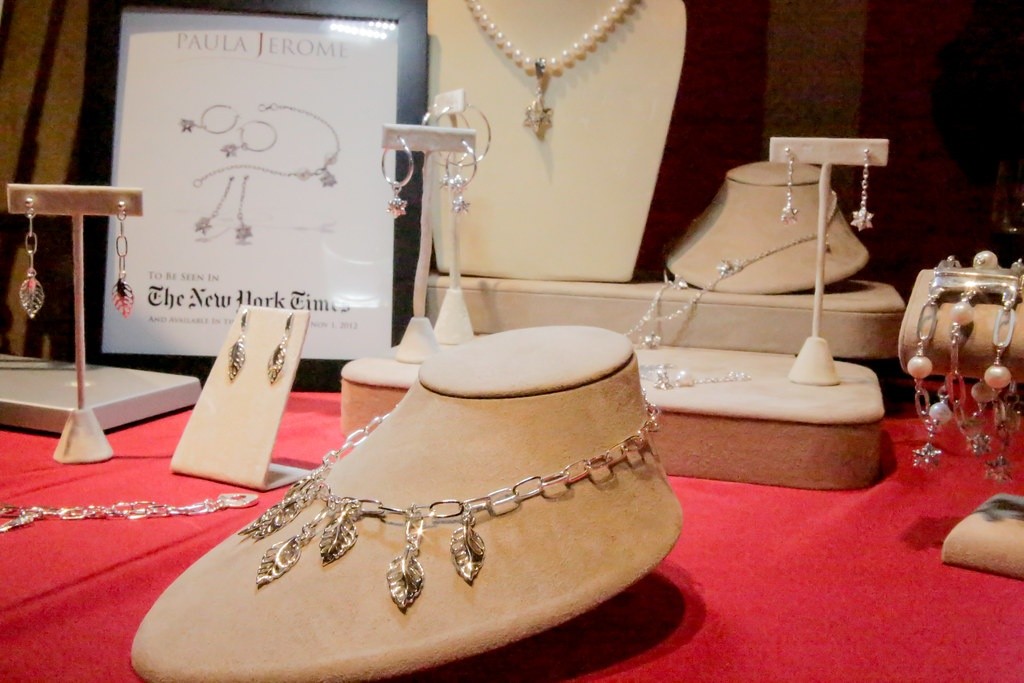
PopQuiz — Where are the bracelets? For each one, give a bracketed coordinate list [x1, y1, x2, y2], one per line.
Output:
[907, 250, 1024, 485]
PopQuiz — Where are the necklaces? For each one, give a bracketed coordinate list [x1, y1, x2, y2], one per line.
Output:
[238, 387, 661, 609]
[466, 0, 635, 135]
[624, 188, 837, 348]
[0, 493, 258, 533]
